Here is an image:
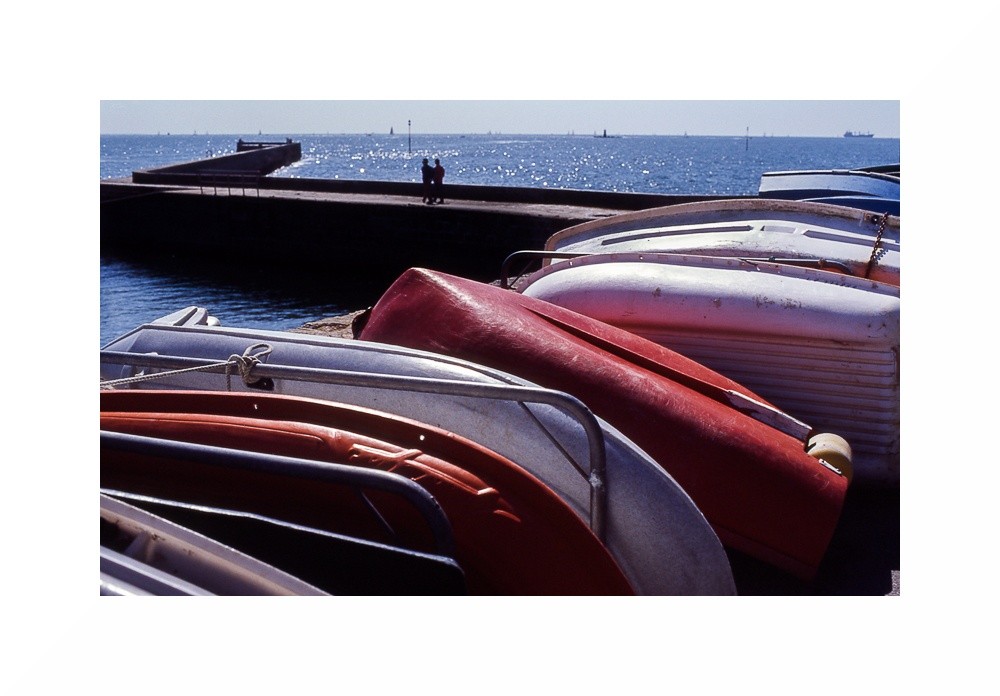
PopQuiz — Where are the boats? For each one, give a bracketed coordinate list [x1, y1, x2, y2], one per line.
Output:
[350, 265, 855, 591]
[507, 250, 900, 492]
[100, 159, 900, 597]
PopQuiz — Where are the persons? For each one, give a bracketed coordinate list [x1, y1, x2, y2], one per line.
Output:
[433, 159, 444, 204]
[420, 158, 436, 204]
